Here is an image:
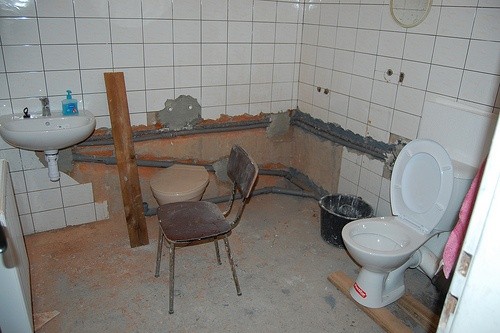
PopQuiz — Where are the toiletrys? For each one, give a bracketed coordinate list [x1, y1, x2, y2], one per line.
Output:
[62, 89, 78, 115]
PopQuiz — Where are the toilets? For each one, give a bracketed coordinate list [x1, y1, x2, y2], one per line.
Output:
[340, 138, 476, 309]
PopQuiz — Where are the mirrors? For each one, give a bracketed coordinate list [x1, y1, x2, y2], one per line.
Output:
[390, 0, 433, 28]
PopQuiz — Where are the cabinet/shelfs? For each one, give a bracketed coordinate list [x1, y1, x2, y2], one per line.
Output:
[0, 159, 34, 333]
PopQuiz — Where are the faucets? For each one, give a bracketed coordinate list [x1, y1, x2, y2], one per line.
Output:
[40, 97, 50, 116]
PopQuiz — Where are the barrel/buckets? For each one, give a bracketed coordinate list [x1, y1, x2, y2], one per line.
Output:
[318, 193, 374, 250]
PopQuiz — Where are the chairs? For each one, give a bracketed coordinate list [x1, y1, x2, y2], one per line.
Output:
[155, 144, 258, 314]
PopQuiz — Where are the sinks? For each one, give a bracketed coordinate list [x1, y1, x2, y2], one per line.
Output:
[0, 111, 96, 152]
